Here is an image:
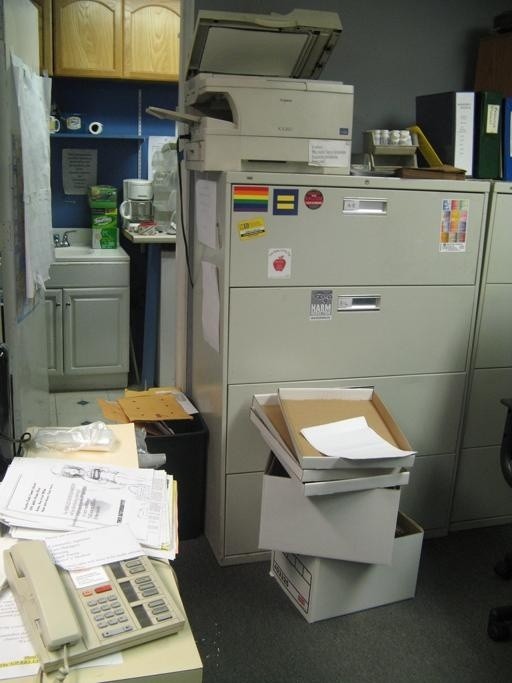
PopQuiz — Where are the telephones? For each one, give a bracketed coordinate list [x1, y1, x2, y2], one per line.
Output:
[3, 524, 186, 675]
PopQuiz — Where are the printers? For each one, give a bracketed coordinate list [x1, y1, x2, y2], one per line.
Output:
[146, 9, 355, 172]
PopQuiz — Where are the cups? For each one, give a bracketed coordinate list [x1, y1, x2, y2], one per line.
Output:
[138, 219, 156, 234]
[50, 115, 61, 133]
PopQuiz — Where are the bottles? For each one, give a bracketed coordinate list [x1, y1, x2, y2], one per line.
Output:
[380, 129, 389, 144]
[391, 130, 400, 144]
[375, 129, 381, 145]
[400, 130, 412, 145]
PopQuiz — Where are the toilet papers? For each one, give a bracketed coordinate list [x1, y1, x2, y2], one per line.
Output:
[88, 121, 104, 135]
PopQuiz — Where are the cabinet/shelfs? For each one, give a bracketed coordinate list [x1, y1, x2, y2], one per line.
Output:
[49, 0, 182, 81]
[198, 171, 492, 565]
[39, 285, 129, 391]
[448, 184, 512, 530]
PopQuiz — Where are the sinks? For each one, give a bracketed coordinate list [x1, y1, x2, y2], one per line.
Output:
[55, 247, 94, 257]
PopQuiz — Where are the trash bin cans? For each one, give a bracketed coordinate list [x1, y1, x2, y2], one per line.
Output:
[144, 394, 209, 541]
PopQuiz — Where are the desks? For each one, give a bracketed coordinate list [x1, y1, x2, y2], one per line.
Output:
[0, 420, 203, 683]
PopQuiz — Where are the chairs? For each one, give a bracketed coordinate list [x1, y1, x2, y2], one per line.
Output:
[486, 395, 512, 642]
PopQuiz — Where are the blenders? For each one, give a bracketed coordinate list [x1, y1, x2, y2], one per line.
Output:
[120, 178, 154, 232]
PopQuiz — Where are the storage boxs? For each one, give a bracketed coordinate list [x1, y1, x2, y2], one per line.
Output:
[252, 446, 401, 564]
[270, 511, 422, 622]
[131, 392, 209, 541]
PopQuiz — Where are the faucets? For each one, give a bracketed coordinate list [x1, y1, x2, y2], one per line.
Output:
[59, 230, 78, 247]
